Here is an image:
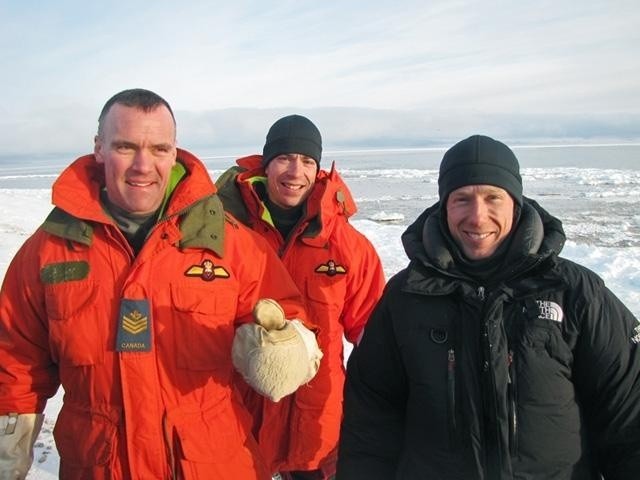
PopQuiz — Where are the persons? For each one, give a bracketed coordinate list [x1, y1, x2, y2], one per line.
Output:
[1, 89, 324, 480]
[216, 114, 387, 480]
[334, 135, 640, 477]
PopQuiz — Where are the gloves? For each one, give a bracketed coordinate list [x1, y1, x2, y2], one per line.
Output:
[231, 298, 323, 404]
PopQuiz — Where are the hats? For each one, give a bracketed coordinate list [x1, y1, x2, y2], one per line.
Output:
[438, 136, 523, 208]
[261, 115, 321, 169]
